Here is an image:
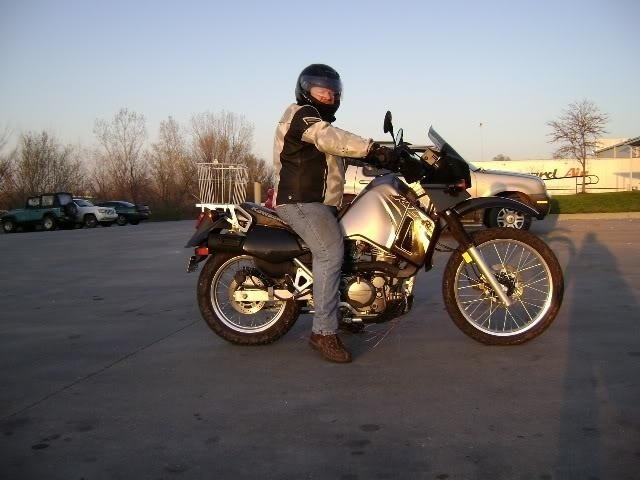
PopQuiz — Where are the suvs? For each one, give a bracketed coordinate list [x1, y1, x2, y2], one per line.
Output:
[333, 133, 553, 241]
[1, 191, 78, 233]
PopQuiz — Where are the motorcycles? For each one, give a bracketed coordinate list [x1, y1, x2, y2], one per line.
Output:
[182, 106, 570, 357]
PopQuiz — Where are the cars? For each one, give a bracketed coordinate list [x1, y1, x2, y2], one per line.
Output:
[0, 197, 152, 234]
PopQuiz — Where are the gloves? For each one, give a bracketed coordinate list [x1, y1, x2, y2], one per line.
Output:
[367, 145, 410, 171]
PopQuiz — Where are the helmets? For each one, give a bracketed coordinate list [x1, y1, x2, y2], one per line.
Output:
[295, 64, 343, 116]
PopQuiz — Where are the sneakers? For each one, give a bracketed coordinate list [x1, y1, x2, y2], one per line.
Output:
[308, 331, 353, 363]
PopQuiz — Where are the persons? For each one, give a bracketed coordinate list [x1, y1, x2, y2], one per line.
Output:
[270, 61, 410, 364]
